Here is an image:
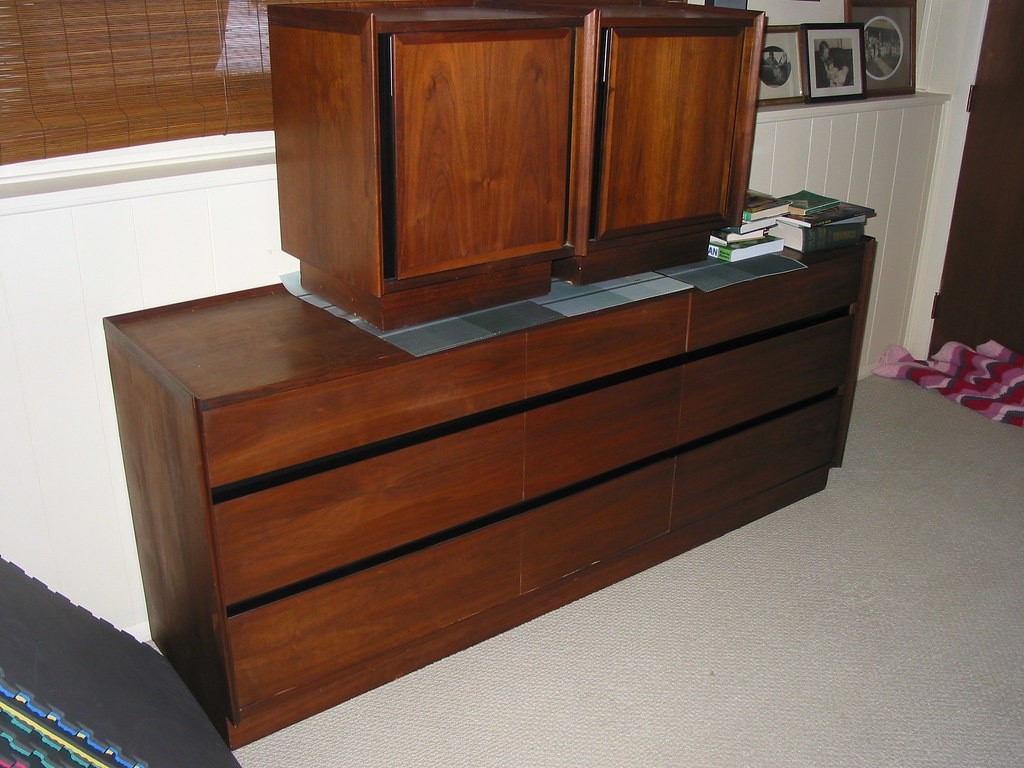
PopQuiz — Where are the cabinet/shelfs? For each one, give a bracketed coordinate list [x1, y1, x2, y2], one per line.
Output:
[266, 0, 767, 339]
[106, 191, 879, 753]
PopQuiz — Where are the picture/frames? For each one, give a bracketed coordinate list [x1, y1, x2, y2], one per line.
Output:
[844, 0, 916, 98]
[802, 22, 869, 103]
[759, 24, 805, 106]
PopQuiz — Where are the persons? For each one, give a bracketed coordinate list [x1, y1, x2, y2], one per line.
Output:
[766, 51, 786, 86]
[816, 40, 853, 88]
[865, 35, 900, 62]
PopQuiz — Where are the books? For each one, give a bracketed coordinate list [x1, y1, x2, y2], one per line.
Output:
[707, 190, 875, 262]
